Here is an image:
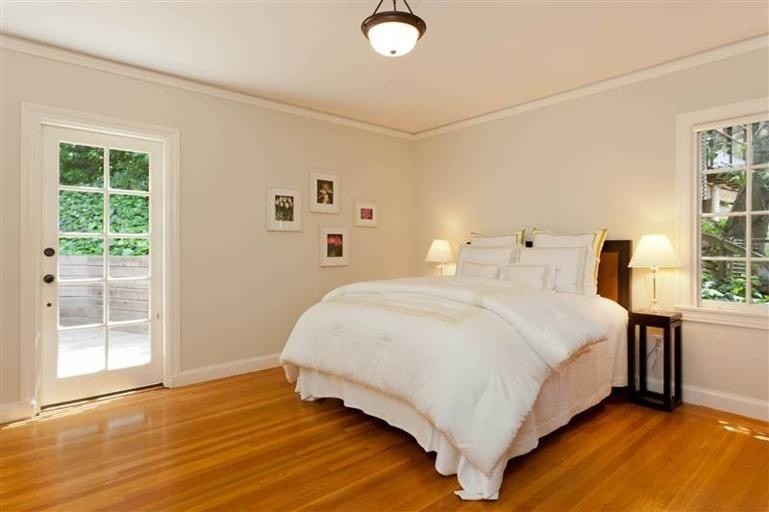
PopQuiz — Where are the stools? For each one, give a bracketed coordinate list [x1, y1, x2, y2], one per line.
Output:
[627, 306, 682, 413]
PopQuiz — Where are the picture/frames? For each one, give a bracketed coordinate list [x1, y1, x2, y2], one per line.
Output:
[353, 200, 378, 227]
[309, 167, 340, 214]
[265, 184, 301, 231]
[319, 225, 349, 266]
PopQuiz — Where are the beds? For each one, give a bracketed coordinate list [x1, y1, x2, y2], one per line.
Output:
[278, 240, 631, 502]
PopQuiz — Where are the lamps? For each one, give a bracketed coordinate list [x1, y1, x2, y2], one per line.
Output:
[627, 232, 684, 313]
[424, 239, 455, 276]
[361, 0, 427, 58]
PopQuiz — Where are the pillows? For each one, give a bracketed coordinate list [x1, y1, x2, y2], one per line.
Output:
[532, 228, 608, 294]
[501, 263, 555, 293]
[458, 245, 513, 279]
[514, 245, 587, 295]
[470, 228, 525, 251]
[460, 261, 499, 279]
